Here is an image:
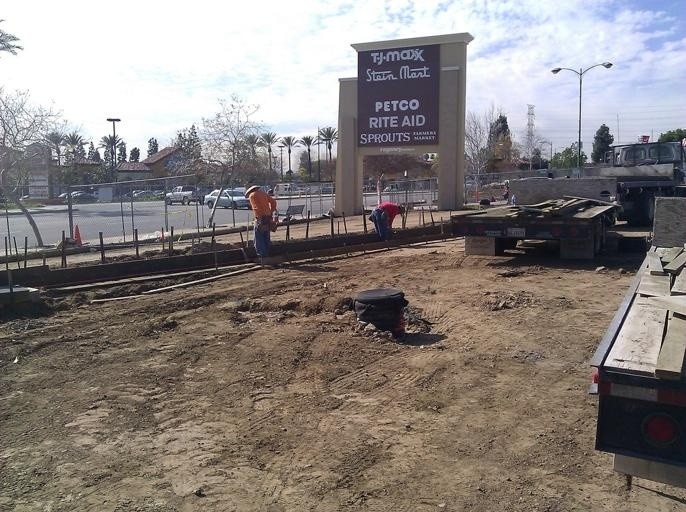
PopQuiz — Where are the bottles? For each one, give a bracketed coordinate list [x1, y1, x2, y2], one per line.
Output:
[511, 192, 516, 205]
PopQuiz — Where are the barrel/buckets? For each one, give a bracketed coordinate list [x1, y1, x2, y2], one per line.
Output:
[355, 289, 407, 332]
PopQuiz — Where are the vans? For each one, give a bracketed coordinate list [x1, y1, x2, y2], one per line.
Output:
[273, 184, 305, 196]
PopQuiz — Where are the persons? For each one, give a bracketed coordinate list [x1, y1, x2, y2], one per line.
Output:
[372, 201, 406, 241]
[244, 185, 277, 268]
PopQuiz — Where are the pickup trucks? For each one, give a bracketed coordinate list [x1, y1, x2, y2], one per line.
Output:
[164, 185, 206, 204]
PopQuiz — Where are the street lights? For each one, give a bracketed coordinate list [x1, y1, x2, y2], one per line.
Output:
[107, 118, 121, 178]
[552, 62, 613, 180]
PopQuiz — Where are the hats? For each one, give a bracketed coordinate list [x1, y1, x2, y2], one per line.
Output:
[400, 207, 405, 216]
[244, 186, 260, 198]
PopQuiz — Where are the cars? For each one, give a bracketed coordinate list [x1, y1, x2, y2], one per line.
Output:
[383, 187, 391, 191]
[58, 191, 94, 199]
[125, 190, 165, 198]
[204, 190, 252, 209]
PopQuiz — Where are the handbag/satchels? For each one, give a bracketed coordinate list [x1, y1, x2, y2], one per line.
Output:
[381, 212, 387, 221]
